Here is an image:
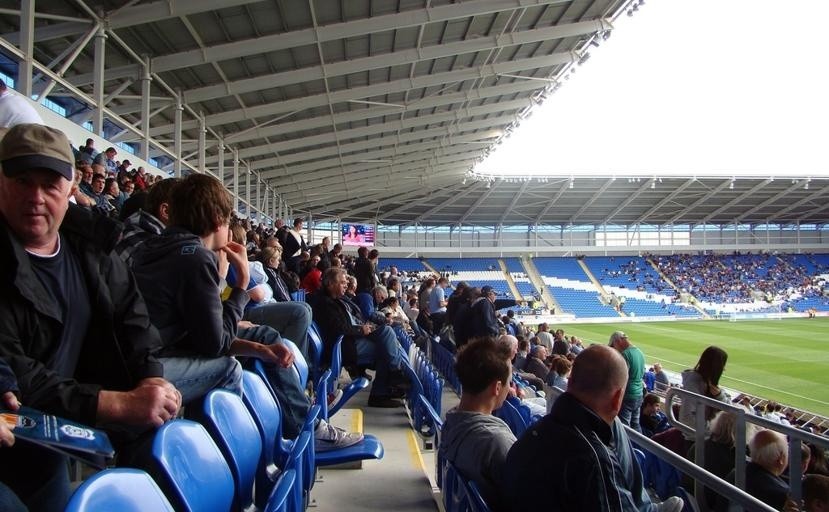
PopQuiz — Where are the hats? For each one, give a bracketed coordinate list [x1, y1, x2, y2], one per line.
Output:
[123, 159, 132, 165]
[607, 331, 628, 346]
[481, 285, 498, 294]
[0, 123, 76, 181]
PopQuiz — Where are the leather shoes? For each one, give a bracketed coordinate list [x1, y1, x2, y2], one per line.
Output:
[362, 372, 372, 381]
[367, 399, 401, 408]
[384, 375, 412, 391]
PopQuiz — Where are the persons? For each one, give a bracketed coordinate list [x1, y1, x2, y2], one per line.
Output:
[1, 78, 829, 511]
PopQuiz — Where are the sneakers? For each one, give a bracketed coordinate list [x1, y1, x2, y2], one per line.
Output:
[327, 388, 344, 410]
[314, 418, 364, 452]
[656, 496, 684, 511]
